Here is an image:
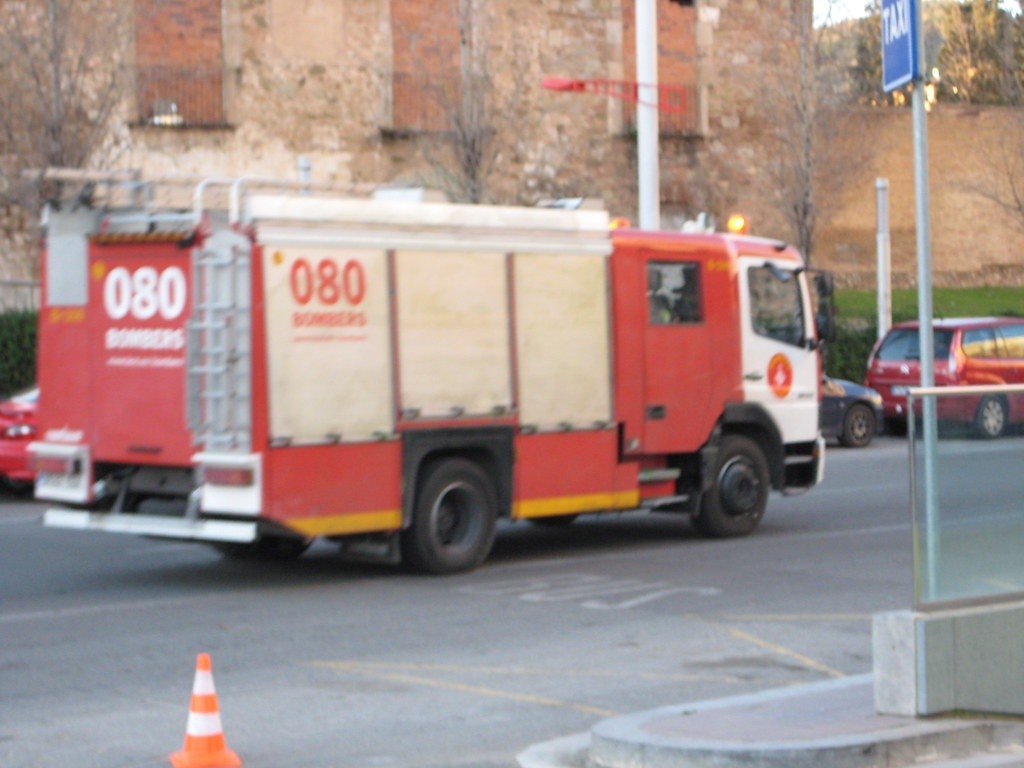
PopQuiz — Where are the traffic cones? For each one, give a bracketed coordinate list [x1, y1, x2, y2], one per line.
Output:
[167, 650, 238, 767]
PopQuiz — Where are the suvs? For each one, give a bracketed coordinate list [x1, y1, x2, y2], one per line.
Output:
[865, 313, 1023, 441]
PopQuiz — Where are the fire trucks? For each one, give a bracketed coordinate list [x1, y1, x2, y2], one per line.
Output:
[18, 163, 836, 581]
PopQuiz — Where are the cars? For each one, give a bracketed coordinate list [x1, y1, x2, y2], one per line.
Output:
[0, 385, 40, 495]
[820, 374, 885, 448]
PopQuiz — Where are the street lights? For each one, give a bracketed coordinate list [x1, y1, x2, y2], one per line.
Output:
[542, 64, 687, 228]
